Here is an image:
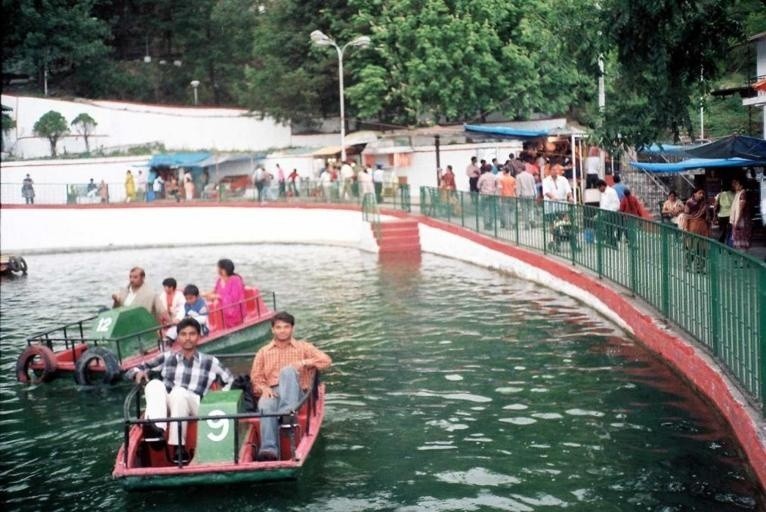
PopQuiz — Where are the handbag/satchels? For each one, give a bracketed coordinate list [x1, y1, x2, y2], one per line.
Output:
[231, 375, 256, 412]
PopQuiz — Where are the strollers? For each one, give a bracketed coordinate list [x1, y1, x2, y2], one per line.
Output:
[549, 198, 582, 255]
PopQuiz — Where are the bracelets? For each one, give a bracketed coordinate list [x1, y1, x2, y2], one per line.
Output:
[300, 360, 307, 367]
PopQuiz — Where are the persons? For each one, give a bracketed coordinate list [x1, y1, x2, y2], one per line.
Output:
[122, 165, 209, 201]
[466, 151, 565, 230]
[592, 180, 620, 251]
[86, 178, 98, 197]
[124, 317, 231, 465]
[618, 189, 644, 249]
[21, 174, 35, 204]
[584, 155, 600, 188]
[165, 284, 210, 344]
[655, 165, 754, 276]
[159, 278, 185, 325]
[252, 159, 383, 202]
[437, 166, 459, 216]
[611, 175, 630, 243]
[542, 162, 575, 235]
[250, 311, 332, 462]
[209, 258, 247, 330]
[98, 267, 155, 317]
[96, 179, 107, 206]
[555, 213, 572, 226]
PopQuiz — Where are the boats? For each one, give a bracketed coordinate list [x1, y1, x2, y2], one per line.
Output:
[110, 351, 325, 486]
[16, 285, 279, 387]
[0, 255, 27, 275]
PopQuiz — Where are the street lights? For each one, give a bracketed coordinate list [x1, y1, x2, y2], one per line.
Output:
[143, 55, 182, 104]
[312, 31, 371, 164]
[43, 16, 97, 97]
[191, 78, 200, 106]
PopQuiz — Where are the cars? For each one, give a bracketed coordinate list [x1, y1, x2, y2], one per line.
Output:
[204, 175, 251, 198]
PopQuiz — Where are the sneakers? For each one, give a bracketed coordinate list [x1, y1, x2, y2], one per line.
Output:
[142, 423, 164, 451]
[260, 449, 276, 461]
[174, 446, 188, 463]
